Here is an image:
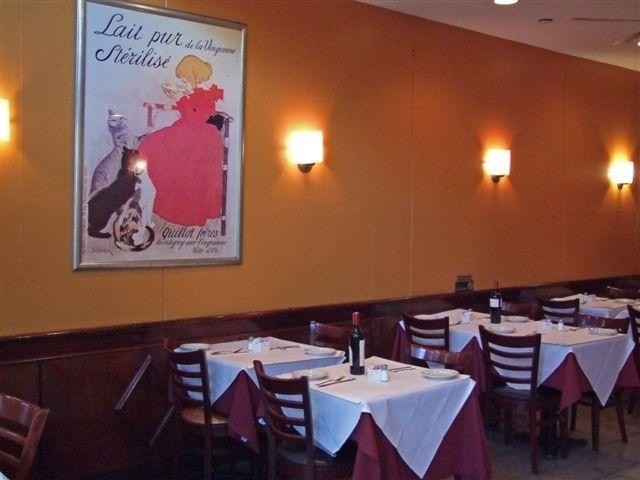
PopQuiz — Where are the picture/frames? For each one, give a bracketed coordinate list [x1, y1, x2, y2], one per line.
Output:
[72, 0, 248, 273]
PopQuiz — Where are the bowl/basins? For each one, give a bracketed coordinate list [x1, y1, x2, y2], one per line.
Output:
[162, 82, 188, 100]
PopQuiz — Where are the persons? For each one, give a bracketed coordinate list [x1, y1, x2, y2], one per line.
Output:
[142, 55, 227, 228]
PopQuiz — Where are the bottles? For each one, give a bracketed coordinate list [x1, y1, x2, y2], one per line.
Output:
[348, 311, 366, 375]
[557, 319, 565, 332]
[248, 336, 254, 350]
[489, 280, 502, 324]
[374, 363, 388, 383]
[583, 291, 597, 304]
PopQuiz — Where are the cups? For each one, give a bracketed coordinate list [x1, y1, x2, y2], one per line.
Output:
[459, 310, 474, 322]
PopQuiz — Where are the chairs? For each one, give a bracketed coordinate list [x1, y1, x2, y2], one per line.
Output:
[625, 304, 640, 416]
[307, 320, 369, 364]
[475, 323, 570, 475]
[402, 314, 452, 375]
[537, 296, 580, 330]
[161, 339, 260, 479]
[252, 360, 358, 479]
[1, 393, 51, 479]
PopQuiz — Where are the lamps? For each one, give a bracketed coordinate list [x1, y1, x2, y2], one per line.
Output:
[284, 127, 327, 175]
[482, 147, 512, 185]
[605, 148, 636, 191]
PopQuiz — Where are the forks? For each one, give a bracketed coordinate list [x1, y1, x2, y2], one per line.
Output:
[316, 376, 344, 387]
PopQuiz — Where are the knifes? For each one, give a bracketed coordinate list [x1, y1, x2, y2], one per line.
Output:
[319, 377, 356, 388]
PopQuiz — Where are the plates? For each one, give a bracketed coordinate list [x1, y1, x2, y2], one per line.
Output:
[290, 369, 326, 380]
[306, 348, 336, 356]
[589, 328, 617, 336]
[419, 368, 461, 379]
[614, 299, 635, 304]
[491, 325, 515, 334]
[180, 343, 211, 351]
[505, 315, 530, 322]
